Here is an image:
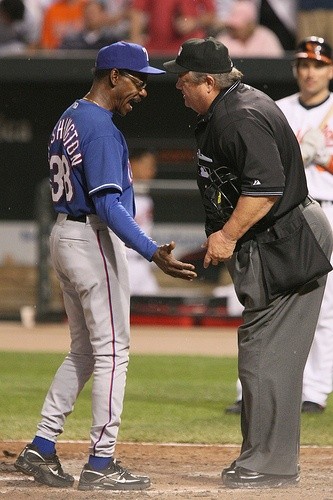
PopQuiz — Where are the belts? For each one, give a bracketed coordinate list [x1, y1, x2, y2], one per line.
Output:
[66, 214, 91, 223]
[302, 195, 315, 207]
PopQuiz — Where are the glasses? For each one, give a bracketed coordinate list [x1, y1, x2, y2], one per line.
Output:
[119, 70, 147, 89]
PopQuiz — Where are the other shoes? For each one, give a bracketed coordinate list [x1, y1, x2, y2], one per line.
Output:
[301, 401, 324, 414]
[225, 401, 243, 415]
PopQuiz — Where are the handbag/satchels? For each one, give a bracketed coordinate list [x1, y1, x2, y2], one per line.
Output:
[257, 206, 333, 300]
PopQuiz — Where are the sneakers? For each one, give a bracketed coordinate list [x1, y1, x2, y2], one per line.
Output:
[78, 458, 151, 491]
[14, 443, 75, 488]
[220, 462, 301, 488]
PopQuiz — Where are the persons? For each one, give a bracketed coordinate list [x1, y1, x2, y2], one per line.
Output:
[22, 0, 284, 59]
[123, 147, 159, 296]
[226, 36, 333, 416]
[162, 35, 333, 489]
[14, 40, 197, 490]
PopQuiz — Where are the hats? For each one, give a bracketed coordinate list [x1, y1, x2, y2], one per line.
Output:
[162, 38, 233, 75]
[292, 36, 333, 64]
[95, 41, 167, 76]
